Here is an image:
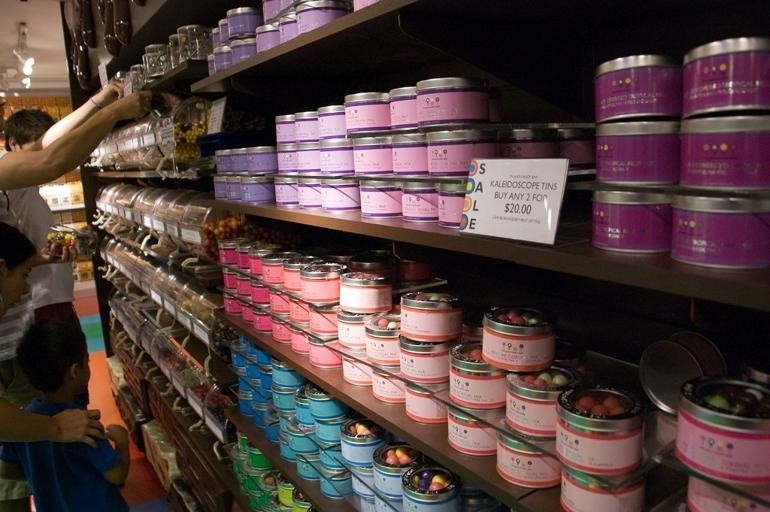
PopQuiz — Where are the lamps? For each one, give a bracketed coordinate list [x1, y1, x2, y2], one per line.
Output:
[12, 44, 36, 69]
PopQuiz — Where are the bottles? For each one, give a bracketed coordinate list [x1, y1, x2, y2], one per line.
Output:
[128, 24, 209, 93]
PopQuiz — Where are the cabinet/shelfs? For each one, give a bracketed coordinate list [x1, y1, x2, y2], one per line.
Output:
[71, 60, 234, 511]
[192, 1, 769, 512]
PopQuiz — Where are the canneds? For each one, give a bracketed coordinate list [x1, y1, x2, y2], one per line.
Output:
[208, 1, 770, 511]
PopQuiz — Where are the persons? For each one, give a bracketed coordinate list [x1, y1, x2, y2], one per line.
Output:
[0, 81, 153, 512]
